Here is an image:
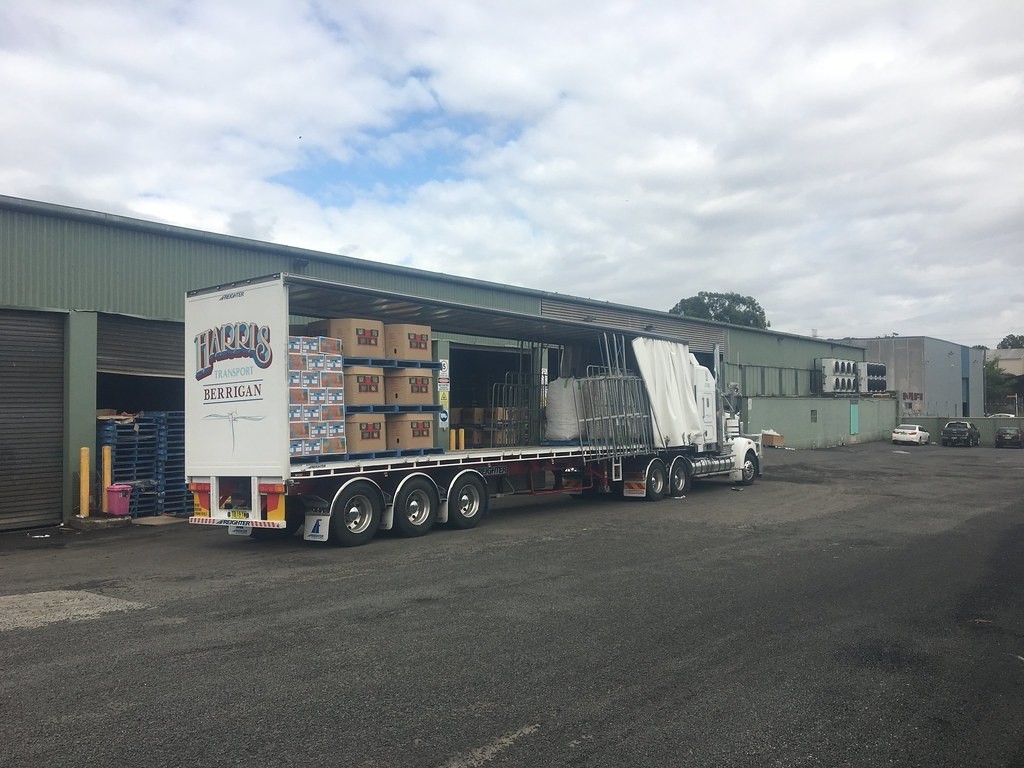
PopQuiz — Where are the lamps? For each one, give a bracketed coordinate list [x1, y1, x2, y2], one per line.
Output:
[288, 257, 309, 270]
[644, 325, 656, 331]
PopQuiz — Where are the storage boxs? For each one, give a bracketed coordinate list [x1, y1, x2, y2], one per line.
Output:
[96, 409, 117, 417]
[450, 406, 525, 444]
[762, 434, 784, 446]
[289, 318, 435, 458]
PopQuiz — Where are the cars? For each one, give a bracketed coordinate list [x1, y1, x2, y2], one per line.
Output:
[995, 426, 1024, 449]
[988, 413, 1015, 418]
[891, 423, 931, 446]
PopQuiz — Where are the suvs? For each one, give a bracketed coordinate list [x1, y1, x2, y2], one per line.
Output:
[941, 420, 981, 447]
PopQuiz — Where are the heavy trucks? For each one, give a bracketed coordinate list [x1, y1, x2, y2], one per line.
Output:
[180, 271, 761, 548]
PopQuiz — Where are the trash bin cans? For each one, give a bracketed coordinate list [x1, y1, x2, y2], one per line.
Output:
[105, 484, 132, 519]
[763, 434, 784, 448]
